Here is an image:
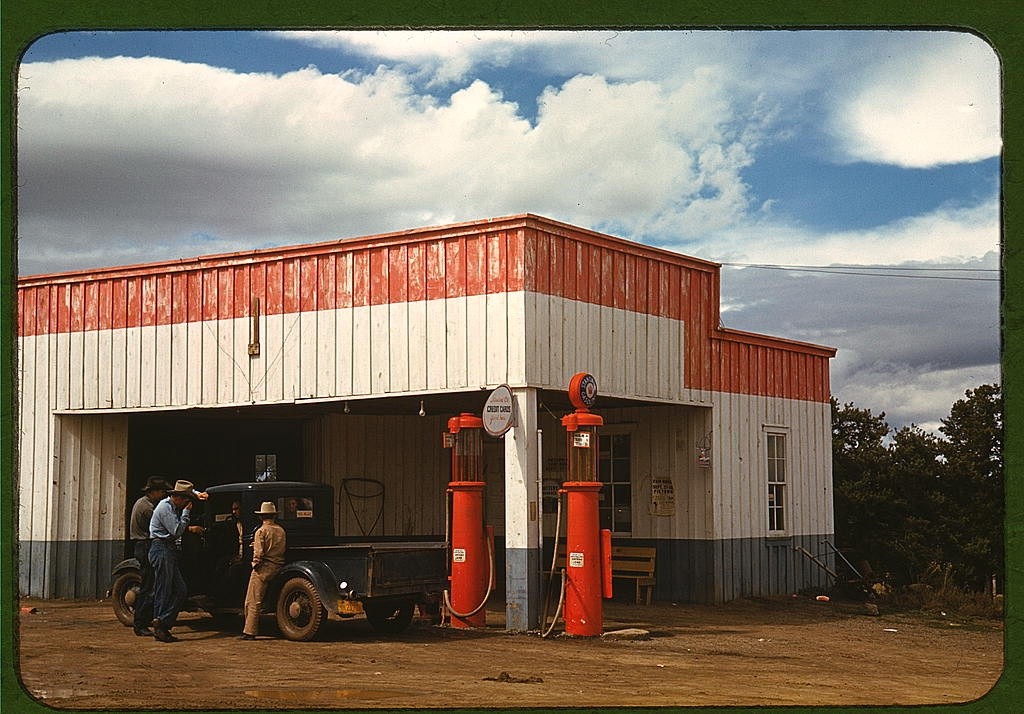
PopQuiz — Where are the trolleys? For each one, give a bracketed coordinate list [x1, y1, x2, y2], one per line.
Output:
[794, 539, 890, 603]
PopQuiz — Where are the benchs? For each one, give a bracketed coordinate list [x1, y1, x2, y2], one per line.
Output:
[544, 543, 657, 605]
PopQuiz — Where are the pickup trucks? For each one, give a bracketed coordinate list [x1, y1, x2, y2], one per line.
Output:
[105, 481, 447, 641]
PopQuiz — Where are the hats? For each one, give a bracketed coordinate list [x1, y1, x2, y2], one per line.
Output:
[167, 480, 200, 502]
[142, 476, 171, 491]
[254, 502, 281, 514]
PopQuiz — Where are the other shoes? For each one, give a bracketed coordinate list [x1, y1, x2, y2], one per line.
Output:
[134, 628, 153, 636]
[154, 630, 178, 643]
[240, 634, 255, 640]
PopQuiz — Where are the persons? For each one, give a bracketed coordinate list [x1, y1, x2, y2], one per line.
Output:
[224, 499, 243, 567]
[240, 501, 286, 640]
[286, 497, 300, 520]
[148, 480, 209, 643]
[129, 477, 205, 636]
